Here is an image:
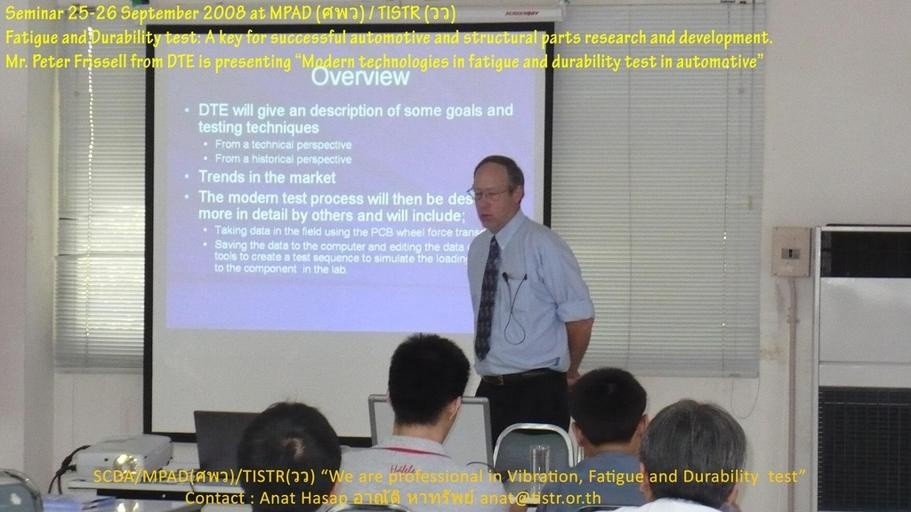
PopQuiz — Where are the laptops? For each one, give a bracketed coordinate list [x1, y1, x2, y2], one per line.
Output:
[194, 410, 261, 479]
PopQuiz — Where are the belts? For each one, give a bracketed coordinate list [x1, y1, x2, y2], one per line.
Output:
[483, 375, 519, 386]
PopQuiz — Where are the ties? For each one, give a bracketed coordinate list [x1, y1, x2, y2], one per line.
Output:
[475, 236, 501, 361]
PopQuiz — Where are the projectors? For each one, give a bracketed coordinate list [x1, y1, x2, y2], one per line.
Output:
[77, 433, 174, 481]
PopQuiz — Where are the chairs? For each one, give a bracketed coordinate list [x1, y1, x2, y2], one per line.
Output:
[0, 422, 741, 512]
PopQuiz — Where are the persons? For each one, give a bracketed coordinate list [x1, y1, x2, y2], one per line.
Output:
[615, 399, 746, 512]
[536, 367, 650, 512]
[468, 156, 595, 455]
[333, 332, 507, 512]
[237, 401, 341, 512]
